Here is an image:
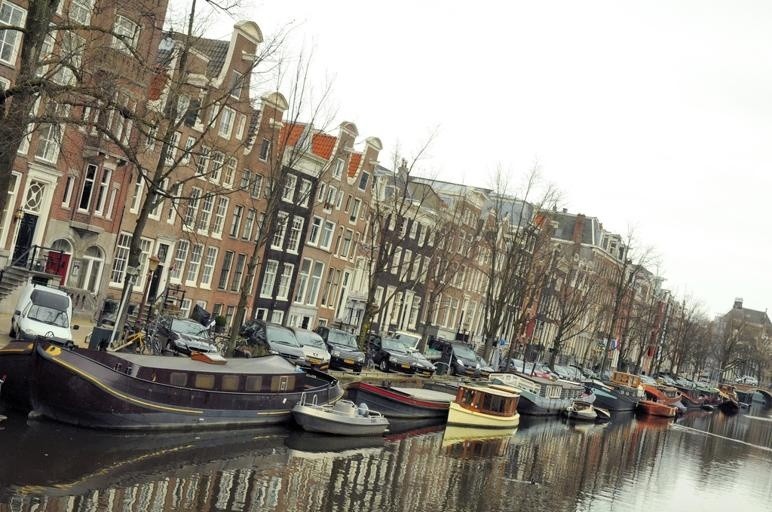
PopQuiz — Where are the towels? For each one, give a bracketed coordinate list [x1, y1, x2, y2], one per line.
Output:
[103, 301, 116, 315]
[127, 304, 136, 315]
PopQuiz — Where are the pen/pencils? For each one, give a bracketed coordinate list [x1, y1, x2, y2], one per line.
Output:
[239, 319, 365, 375]
[366, 330, 496, 378]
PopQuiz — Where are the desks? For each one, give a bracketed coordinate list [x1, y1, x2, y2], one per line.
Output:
[366, 302, 379, 345]
[134, 256, 160, 328]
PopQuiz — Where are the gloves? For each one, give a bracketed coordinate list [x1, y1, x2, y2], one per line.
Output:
[443, 381, 523, 436]
[423, 381, 479, 396]
[293, 391, 390, 437]
[488, 374, 741, 420]
[0, 333, 345, 431]
[344, 378, 457, 434]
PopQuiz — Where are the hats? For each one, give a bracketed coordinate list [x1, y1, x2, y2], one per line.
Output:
[736, 375, 758, 386]
[500, 359, 709, 390]
[145, 314, 219, 358]
